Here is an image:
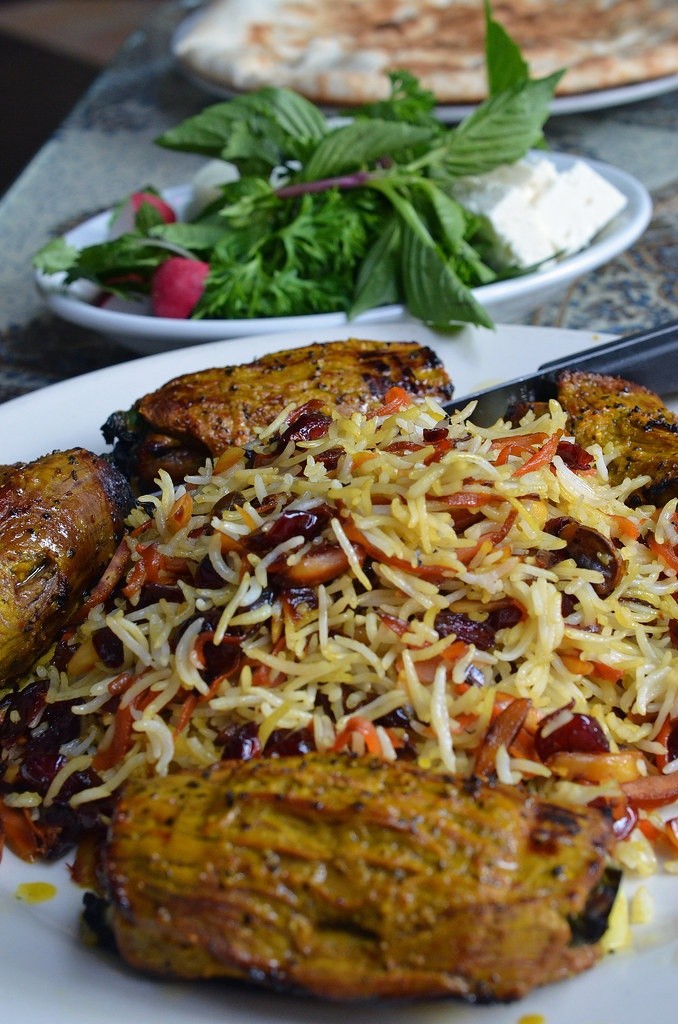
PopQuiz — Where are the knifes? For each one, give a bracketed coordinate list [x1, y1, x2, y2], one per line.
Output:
[133, 316, 677, 508]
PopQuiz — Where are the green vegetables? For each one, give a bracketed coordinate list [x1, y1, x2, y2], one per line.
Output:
[27, 0, 567, 331]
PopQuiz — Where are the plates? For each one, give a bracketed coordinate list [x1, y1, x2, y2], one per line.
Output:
[169, 1, 678, 120]
[1, 317, 678, 1023]
[35, 149, 655, 359]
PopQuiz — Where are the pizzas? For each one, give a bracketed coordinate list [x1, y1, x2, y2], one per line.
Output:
[173, 0, 678, 103]
